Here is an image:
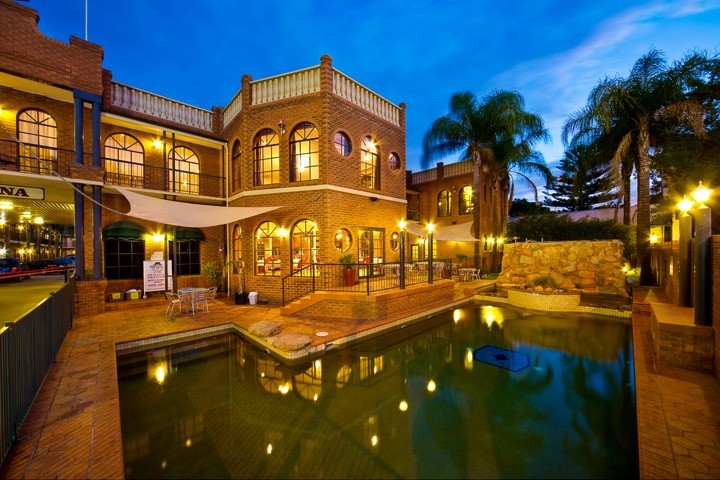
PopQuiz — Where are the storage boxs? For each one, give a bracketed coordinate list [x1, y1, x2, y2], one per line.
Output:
[107, 290, 143, 302]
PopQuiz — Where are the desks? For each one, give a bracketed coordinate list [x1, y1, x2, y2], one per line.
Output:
[458, 266, 477, 282]
[176, 287, 210, 315]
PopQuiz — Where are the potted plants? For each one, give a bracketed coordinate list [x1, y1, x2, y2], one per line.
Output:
[338, 252, 359, 288]
[199, 261, 223, 293]
[222, 259, 247, 305]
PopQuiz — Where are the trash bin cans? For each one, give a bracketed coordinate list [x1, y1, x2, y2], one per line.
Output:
[248, 292, 258, 305]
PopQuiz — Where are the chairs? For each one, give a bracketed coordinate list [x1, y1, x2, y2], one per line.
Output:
[470, 269, 482, 281]
[164, 286, 218, 317]
